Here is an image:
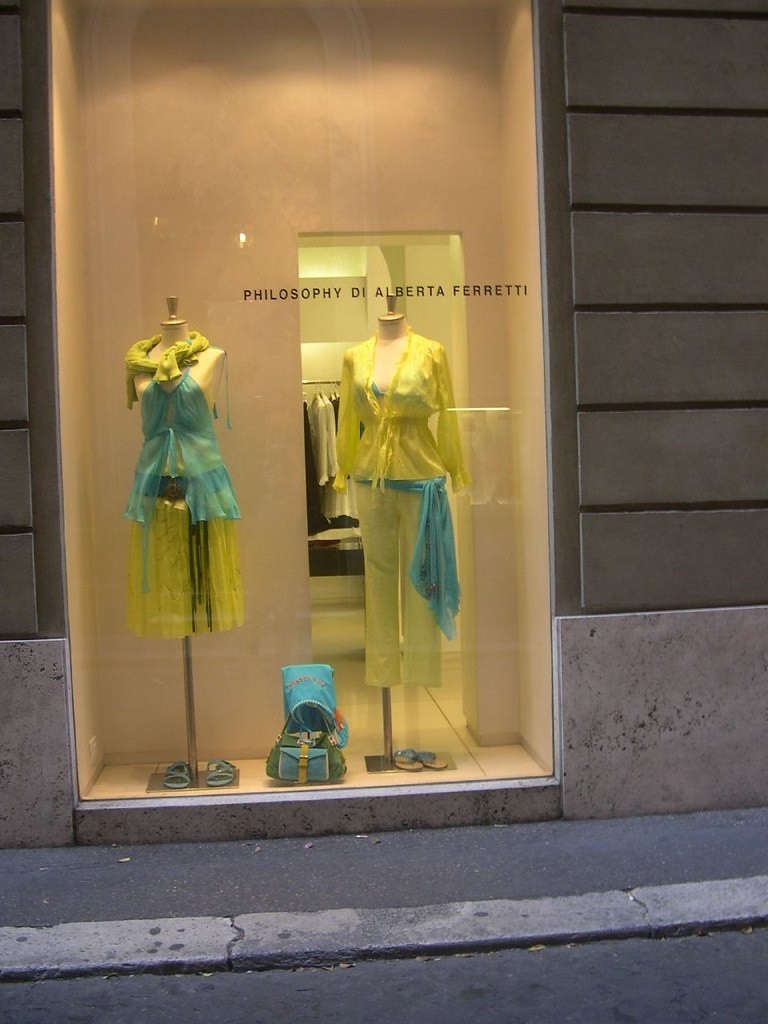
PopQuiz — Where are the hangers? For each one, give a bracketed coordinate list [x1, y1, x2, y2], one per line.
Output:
[300, 380, 340, 409]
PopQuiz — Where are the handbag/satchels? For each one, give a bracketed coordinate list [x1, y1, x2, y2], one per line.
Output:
[266, 706, 347, 782]
[281, 662, 335, 744]
[332, 708, 348, 748]
[279, 745, 329, 782]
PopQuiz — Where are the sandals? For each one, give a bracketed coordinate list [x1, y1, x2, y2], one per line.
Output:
[206, 758, 236, 786]
[164, 760, 191, 789]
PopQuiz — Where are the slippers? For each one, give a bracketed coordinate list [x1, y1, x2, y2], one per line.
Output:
[418, 750, 448, 769]
[395, 749, 423, 771]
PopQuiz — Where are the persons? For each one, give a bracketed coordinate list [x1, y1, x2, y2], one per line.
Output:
[333, 313, 473, 687]
[123, 314, 245, 638]
